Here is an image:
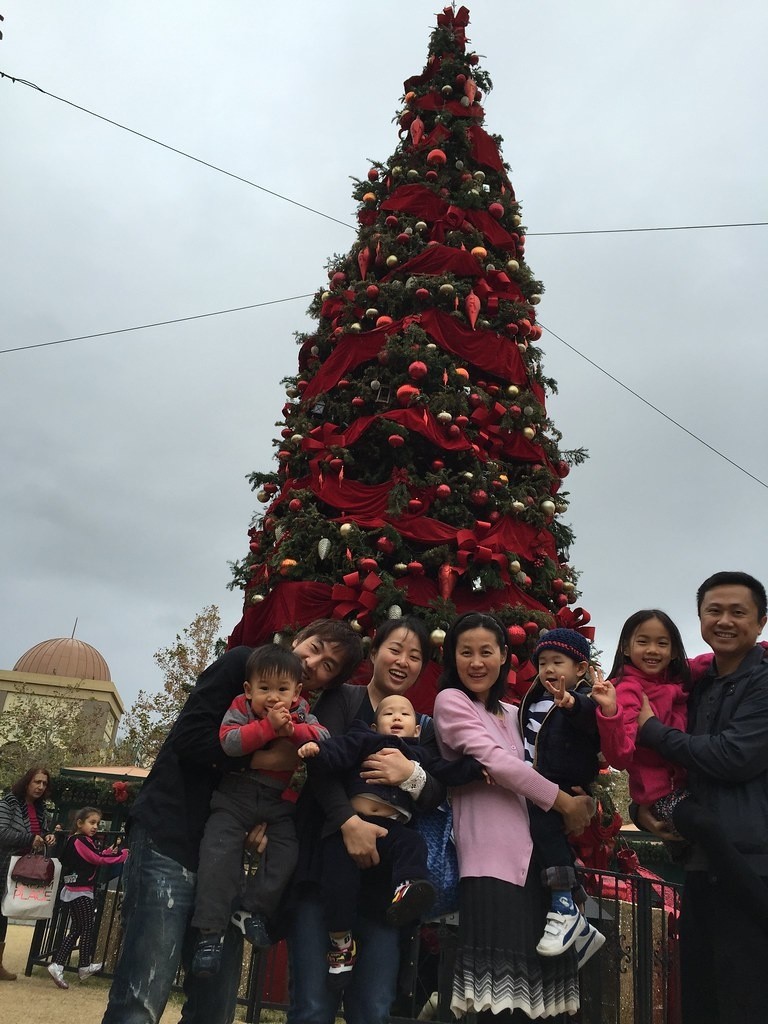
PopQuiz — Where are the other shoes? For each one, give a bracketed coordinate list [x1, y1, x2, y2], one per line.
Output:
[387, 880, 435, 926]
[78, 961, 107, 980]
[326, 940, 360, 992]
[48, 963, 69, 989]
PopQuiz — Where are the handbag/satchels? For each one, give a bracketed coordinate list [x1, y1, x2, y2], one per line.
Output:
[11, 843, 54, 886]
[1, 843, 62, 920]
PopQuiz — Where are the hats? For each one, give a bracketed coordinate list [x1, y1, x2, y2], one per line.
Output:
[532, 628, 590, 668]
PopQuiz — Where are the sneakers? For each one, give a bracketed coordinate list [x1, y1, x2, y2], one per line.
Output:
[229, 909, 271, 949]
[536, 903, 585, 956]
[192, 931, 226, 977]
[575, 924, 606, 969]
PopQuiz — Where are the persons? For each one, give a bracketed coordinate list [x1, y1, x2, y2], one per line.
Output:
[590, 607, 768, 934]
[638, 571, 768, 1024]
[0, 767, 56, 981]
[54, 824, 124, 849]
[47, 806, 130, 989]
[101, 611, 605, 1024]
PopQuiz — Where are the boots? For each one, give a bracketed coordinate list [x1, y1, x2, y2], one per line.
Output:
[0, 942, 17, 980]
